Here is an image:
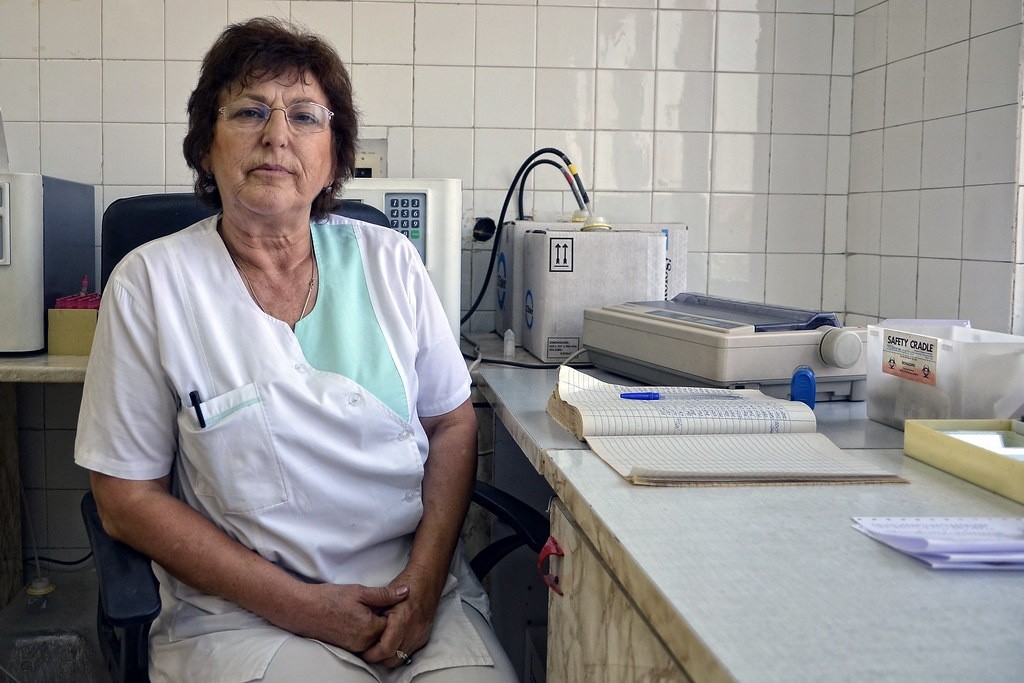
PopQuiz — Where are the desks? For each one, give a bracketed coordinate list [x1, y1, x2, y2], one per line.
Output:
[1, 325, 1024, 683]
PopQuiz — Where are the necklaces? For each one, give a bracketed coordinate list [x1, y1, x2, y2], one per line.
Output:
[225, 242, 316, 323]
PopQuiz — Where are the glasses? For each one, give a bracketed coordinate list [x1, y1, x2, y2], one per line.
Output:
[217, 99, 335, 133]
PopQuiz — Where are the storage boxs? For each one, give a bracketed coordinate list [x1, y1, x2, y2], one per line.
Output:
[864, 317, 1024, 432]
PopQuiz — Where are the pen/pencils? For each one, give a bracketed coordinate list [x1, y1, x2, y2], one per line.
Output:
[189, 389, 209, 429]
[621, 391, 742, 400]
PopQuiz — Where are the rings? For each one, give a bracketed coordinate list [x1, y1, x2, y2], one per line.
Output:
[397, 651, 414, 667]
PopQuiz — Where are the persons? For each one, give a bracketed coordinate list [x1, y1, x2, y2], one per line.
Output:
[72, 17, 520, 683]
